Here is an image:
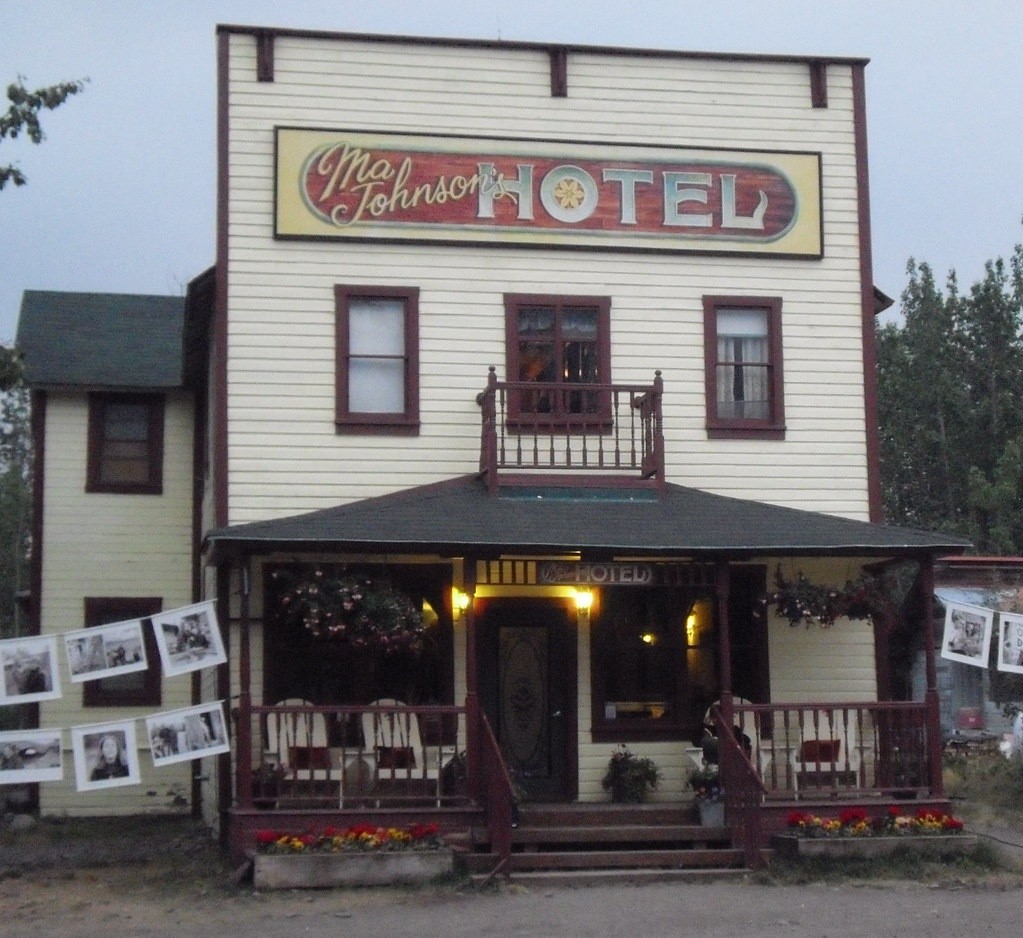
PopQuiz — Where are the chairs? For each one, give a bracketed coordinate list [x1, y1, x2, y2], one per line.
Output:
[362, 698, 438, 779]
[702, 697, 764, 785]
[786, 710, 861, 800]
[267, 698, 342, 780]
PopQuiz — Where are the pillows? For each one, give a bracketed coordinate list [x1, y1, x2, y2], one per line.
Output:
[288, 747, 331, 769]
[377, 746, 418, 770]
[801, 740, 841, 762]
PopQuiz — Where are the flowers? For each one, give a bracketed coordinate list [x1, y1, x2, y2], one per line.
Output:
[253, 822, 440, 854]
[786, 807, 965, 838]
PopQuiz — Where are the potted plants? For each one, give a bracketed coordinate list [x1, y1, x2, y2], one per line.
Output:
[685, 765, 725, 827]
[602, 744, 666, 804]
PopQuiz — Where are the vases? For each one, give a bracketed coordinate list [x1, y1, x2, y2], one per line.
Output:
[253, 848, 455, 890]
[774, 833, 977, 861]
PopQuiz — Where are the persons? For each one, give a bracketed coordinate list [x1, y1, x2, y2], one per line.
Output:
[950, 612, 965, 653]
[692, 704, 752, 764]
[110, 643, 128, 668]
[90, 735, 129, 780]
[11, 652, 45, 694]
[180, 623, 209, 667]
[153, 716, 213, 759]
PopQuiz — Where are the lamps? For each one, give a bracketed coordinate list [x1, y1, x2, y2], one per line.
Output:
[459, 592, 468, 614]
[577, 585, 592, 615]
